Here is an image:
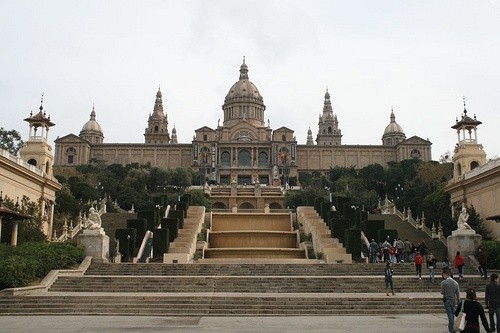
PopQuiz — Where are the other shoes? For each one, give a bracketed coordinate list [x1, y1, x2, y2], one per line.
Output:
[458, 276, 463, 280]
[481, 274, 487, 280]
[398, 260, 404, 263]
[418, 277, 421, 280]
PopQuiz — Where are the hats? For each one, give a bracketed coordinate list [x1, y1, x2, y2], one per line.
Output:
[464, 288, 476, 294]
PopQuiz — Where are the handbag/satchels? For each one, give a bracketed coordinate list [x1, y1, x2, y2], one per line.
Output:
[453, 312, 467, 332]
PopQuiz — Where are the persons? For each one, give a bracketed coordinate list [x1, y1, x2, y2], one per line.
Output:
[485, 274, 500, 333]
[478, 248, 487, 280]
[454, 288, 490, 333]
[369, 238, 427, 263]
[457, 208, 473, 230]
[414, 252, 423, 279]
[384, 263, 395, 296]
[454, 251, 465, 279]
[427, 255, 437, 285]
[86, 207, 102, 229]
[440, 270, 460, 333]
[442, 258, 451, 273]
